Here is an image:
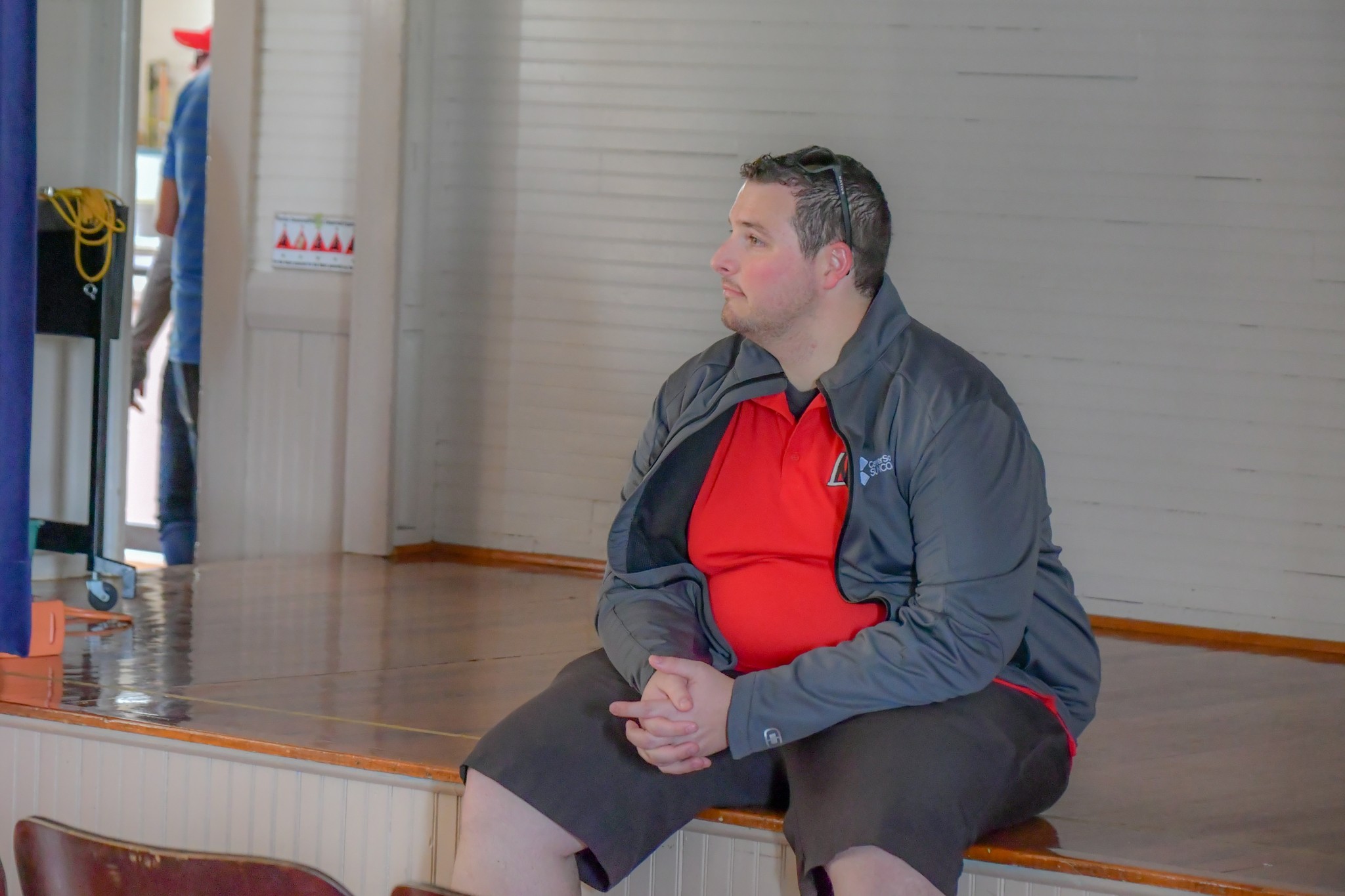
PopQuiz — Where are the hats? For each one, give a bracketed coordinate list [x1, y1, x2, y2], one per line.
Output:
[173, 22, 212, 56]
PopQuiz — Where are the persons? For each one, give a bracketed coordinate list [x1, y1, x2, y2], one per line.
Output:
[172, 23, 213, 74]
[128, 59, 212, 568]
[445, 145, 1104, 896]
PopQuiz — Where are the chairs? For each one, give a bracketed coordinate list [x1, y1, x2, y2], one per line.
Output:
[13, 815, 354, 896]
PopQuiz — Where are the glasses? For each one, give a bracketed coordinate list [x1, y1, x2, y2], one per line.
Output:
[194, 50, 209, 67]
[792, 143, 853, 280]
